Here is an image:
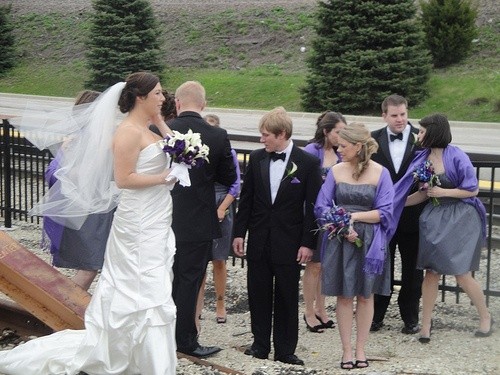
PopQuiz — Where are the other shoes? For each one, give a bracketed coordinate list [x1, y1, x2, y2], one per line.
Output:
[355, 360, 368, 368]
[216, 317, 226, 323]
[340, 361, 354, 369]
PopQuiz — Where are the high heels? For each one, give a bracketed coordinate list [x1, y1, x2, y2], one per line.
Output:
[419, 318, 432, 343]
[303, 313, 324, 333]
[475, 316, 494, 337]
[315, 313, 335, 328]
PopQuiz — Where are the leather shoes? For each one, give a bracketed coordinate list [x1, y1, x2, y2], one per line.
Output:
[276, 354, 304, 365]
[404, 322, 418, 334]
[187, 342, 220, 357]
[244, 349, 259, 357]
[370, 320, 384, 332]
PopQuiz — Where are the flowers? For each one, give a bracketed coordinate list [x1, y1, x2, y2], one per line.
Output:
[413, 162, 441, 205]
[311, 206, 363, 248]
[410, 133, 419, 153]
[281, 162, 299, 183]
[163, 129, 210, 192]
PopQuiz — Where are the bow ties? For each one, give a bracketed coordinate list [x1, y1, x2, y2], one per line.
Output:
[269, 151, 286, 161]
[390, 132, 403, 141]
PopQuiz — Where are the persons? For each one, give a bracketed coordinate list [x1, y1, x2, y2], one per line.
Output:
[402, 113, 495, 345]
[234, 106, 322, 364]
[368, 95, 423, 333]
[45, 89, 116, 292]
[312, 123, 395, 370]
[303, 110, 348, 334]
[197, 114, 242, 322]
[0, 72, 192, 375]
[149, 90, 178, 139]
[149, 81, 238, 356]
